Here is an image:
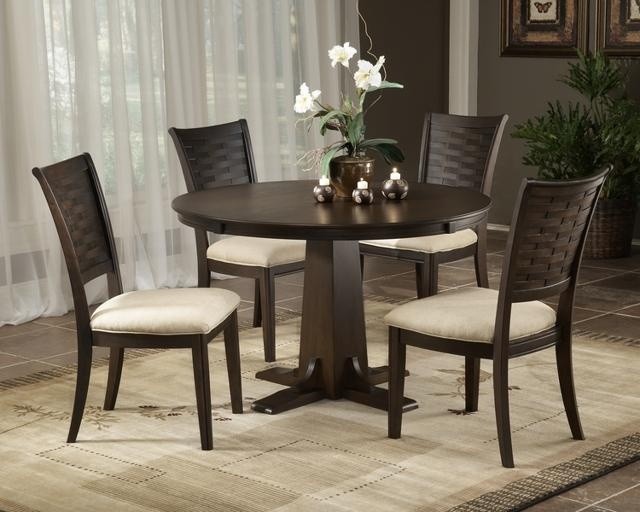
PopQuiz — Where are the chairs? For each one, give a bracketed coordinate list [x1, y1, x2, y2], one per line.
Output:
[168, 119, 306, 363]
[30, 152, 242, 450]
[383, 164, 614, 466]
[360, 113, 509, 302]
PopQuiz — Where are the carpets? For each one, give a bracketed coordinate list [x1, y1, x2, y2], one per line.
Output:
[0, 294, 639, 512]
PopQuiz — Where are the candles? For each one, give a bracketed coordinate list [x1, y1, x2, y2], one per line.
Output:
[357, 177, 369, 190]
[319, 175, 329, 186]
[389, 167, 401, 180]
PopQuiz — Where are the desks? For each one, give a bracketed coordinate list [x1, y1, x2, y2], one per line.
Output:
[172, 179, 494, 415]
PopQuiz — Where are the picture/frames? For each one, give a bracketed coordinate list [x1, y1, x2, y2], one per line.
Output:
[498, 0, 588, 59]
[594, 1, 640, 59]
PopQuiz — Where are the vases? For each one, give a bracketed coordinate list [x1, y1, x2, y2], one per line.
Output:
[328, 156, 376, 198]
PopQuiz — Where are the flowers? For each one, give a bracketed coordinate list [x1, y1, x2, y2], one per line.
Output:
[290, 0, 406, 177]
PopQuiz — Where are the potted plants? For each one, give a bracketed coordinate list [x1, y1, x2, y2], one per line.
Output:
[508, 46, 640, 261]
